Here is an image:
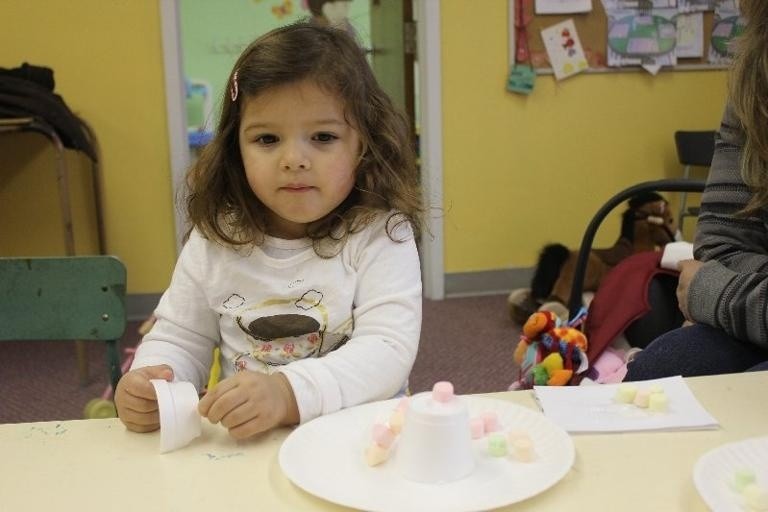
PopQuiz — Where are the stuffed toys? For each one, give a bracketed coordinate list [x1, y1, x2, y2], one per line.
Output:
[514, 301, 591, 390]
[506, 192, 682, 321]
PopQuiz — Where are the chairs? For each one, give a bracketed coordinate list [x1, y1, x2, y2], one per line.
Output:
[0, 254, 127, 390]
[672, 130, 716, 234]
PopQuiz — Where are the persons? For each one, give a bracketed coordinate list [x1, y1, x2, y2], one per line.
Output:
[607, 0, 768, 382]
[115, 22, 422, 444]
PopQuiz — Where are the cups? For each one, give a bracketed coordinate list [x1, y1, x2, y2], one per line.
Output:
[149, 378, 203, 453]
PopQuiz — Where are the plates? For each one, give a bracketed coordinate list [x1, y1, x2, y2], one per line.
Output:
[277, 394, 574, 511]
[694, 435, 768, 511]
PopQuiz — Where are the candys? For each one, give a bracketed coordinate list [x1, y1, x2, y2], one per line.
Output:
[432, 380, 455, 401]
[612, 381, 671, 414]
[363, 395, 410, 468]
[732, 464, 768, 511]
[468, 410, 535, 462]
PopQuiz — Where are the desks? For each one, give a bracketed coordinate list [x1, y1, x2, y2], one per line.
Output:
[0, 372, 768, 512]
